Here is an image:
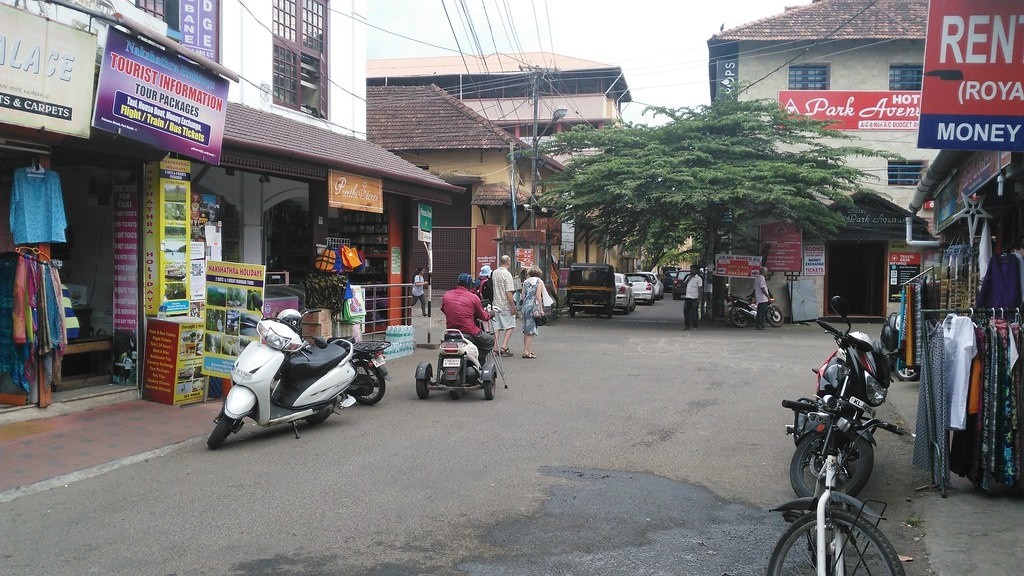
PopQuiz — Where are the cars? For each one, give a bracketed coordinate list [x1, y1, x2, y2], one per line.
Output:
[614, 272, 664, 314]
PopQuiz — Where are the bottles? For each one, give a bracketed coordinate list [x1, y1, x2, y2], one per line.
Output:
[382, 325, 414, 360]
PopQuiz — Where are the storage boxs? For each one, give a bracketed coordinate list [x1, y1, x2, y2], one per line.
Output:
[300, 308, 333, 346]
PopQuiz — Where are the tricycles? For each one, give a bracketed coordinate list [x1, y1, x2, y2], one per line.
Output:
[567, 263, 616, 319]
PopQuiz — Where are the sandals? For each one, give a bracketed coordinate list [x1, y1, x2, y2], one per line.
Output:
[493, 350, 503, 356]
[522, 351, 536, 358]
[500, 346, 513, 356]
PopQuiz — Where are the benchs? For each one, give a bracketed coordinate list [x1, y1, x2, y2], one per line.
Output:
[50, 334, 114, 391]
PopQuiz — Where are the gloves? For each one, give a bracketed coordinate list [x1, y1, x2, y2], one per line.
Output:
[489, 310, 495, 318]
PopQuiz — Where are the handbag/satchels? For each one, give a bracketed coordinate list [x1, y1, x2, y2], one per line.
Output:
[535, 314, 547, 326]
[481, 270, 494, 301]
[337, 291, 367, 326]
[315, 245, 365, 274]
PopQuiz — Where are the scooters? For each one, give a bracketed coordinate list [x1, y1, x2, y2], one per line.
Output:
[207, 292, 358, 450]
[415, 303, 502, 400]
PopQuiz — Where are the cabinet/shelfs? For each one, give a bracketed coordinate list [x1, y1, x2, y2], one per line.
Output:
[330, 193, 404, 331]
[272, 202, 312, 283]
[927, 307, 1024, 329]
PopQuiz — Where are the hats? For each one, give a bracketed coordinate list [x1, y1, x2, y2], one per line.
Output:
[479, 265, 492, 276]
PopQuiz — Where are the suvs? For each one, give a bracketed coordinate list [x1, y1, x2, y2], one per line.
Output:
[663, 269, 703, 300]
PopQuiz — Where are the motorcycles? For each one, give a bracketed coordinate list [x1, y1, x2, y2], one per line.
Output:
[782, 296, 919, 498]
[275, 307, 391, 405]
[725, 288, 784, 328]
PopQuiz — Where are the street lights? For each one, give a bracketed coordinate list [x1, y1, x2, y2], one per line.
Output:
[529, 107, 567, 229]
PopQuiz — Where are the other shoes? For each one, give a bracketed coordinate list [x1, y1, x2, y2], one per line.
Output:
[692, 325, 698, 330]
[681, 327, 690, 330]
[756, 327, 765, 330]
[422, 313, 428, 317]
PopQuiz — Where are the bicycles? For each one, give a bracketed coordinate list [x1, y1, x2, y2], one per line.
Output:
[766, 395, 916, 576]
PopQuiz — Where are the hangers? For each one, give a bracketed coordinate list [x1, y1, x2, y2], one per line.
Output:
[14, 243, 52, 263]
[26, 154, 45, 178]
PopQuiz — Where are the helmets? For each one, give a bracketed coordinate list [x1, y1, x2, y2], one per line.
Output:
[276, 309, 302, 327]
[458, 273, 472, 287]
[837, 332, 873, 363]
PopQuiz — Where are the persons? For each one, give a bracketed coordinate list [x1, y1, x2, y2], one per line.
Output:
[753, 267, 771, 330]
[520, 265, 544, 358]
[682, 265, 703, 331]
[441, 273, 495, 366]
[412, 267, 428, 317]
[120, 347, 134, 384]
[473, 265, 492, 300]
[491, 254, 518, 355]
